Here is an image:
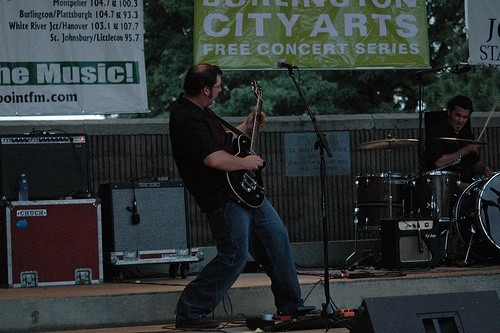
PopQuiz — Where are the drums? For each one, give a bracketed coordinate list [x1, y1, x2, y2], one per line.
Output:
[407, 170, 464, 224]
[452, 170, 500, 259]
[353, 172, 411, 232]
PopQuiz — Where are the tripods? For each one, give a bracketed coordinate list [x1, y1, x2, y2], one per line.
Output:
[282, 69, 349, 333]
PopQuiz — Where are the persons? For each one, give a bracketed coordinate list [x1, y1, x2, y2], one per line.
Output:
[419, 95, 493, 236]
[170, 62, 322, 331]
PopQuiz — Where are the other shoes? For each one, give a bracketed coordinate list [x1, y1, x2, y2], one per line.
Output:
[176, 319, 220, 328]
[276, 303, 317, 317]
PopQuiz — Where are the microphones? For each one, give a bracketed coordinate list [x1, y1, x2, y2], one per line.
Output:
[131, 200, 140, 225]
[417, 224, 423, 253]
[277, 58, 298, 69]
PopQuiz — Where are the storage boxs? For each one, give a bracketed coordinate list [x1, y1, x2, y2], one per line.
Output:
[6, 199, 104, 288]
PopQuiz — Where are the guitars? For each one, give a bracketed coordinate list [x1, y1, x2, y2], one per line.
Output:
[225, 80, 266, 209]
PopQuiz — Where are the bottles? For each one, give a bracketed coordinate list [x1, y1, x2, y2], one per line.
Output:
[19, 174, 29, 201]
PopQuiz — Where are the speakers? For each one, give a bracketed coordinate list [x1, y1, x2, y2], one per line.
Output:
[98, 179, 192, 253]
[350, 290, 500, 333]
[0, 132, 92, 200]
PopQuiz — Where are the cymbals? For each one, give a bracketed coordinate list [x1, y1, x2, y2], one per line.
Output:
[420, 65, 472, 75]
[350, 138, 420, 151]
[435, 137, 490, 145]
